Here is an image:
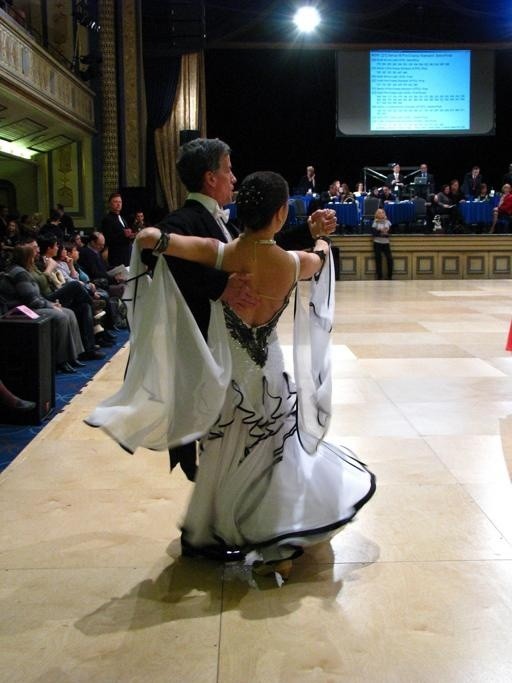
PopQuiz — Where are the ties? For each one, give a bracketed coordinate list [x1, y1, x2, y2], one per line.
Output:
[423, 173, 426, 178]
[473, 177, 476, 185]
[97, 254, 106, 273]
[118, 215, 126, 229]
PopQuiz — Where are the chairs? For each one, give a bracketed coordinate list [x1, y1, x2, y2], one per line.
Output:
[296, 201, 308, 224]
[412, 198, 426, 228]
[359, 196, 381, 234]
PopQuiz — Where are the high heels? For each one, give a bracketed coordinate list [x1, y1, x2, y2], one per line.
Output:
[270, 559, 292, 580]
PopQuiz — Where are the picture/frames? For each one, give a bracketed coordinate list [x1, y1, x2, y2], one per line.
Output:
[42, 0, 75, 66]
[48, 142, 83, 217]
[27, 135, 74, 153]
[0, 117, 47, 142]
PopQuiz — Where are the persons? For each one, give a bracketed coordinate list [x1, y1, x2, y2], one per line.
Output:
[434, 185, 465, 229]
[379, 186, 396, 207]
[40, 234, 116, 347]
[1, 380, 36, 411]
[415, 164, 434, 184]
[353, 183, 368, 200]
[371, 209, 394, 280]
[450, 181, 467, 207]
[367, 186, 381, 200]
[79, 232, 125, 287]
[102, 194, 136, 268]
[339, 184, 354, 203]
[320, 184, 338, 209]
[40, 210, 64, 239]
[385, 163, 408, 201]
[299, 166, 318, 194]
[54, 243, 85, 286]
[479, 184, 488, 200]
[490, 184, 512, 234]
[141, 137, 261, 485]
[461, 167, 483, 200]
[25, 239, 107, 360]
[334, 180, 341, 196]
[57, 204, 75, 234]
[112, 172, 377, 579]
[134, 211, 145, 232]
[0, 245, 86, 373]
[0, 221, 22, 261]
[65, 244, 102, 298]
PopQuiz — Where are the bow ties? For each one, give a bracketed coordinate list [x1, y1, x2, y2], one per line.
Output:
[209, 201, 232, 225]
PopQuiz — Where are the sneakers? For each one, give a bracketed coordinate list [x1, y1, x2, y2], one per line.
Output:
[113, 325, 120, 331]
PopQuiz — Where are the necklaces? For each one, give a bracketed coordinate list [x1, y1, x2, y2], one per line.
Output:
[238, 232, 276, 263]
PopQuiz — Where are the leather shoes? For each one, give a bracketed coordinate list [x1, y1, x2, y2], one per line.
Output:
[62, 361, 77, 374]
[107, 330, 118, 338]
[99, 338, 112, 347]
[92, 350, 107, 359]
[73, 359, 87, 367]
[111, 329, 119, 335]
[92, 299, 107, 309]
[6, 397, 36, 411]
[93, 343, 101, 349]
